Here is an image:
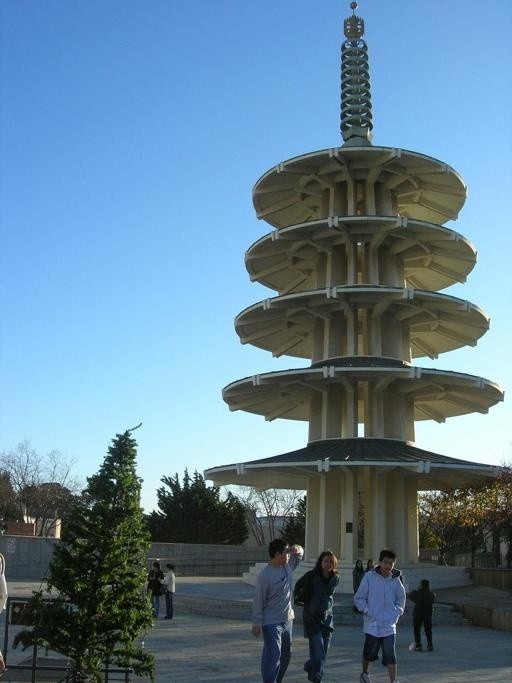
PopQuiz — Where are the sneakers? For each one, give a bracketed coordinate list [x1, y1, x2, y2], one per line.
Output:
[390, 679, 399, 683]
[165, 615, 173, 621]
[151, 614, 159, 618]
[415, 643, 423, 650]
[359, 672, 371, 683]
[427, 643, 434, 652]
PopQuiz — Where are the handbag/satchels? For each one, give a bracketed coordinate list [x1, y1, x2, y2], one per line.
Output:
[160, 580, 168, 595]
[0, 553, 8, 614]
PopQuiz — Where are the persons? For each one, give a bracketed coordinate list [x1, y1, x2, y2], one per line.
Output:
[157, 563, 177, 620]
[352, 547, 408, 682]
[364, 559, 372, 570]
[351, 558, 366, 614]
[295, 548, 341, 682]
[144, 562, 163, 619]
[251, 538, 307, 682]
[406, 577, 437, 652]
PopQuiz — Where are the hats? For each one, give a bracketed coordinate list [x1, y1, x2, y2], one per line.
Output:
[152, 561, 161, 568]
